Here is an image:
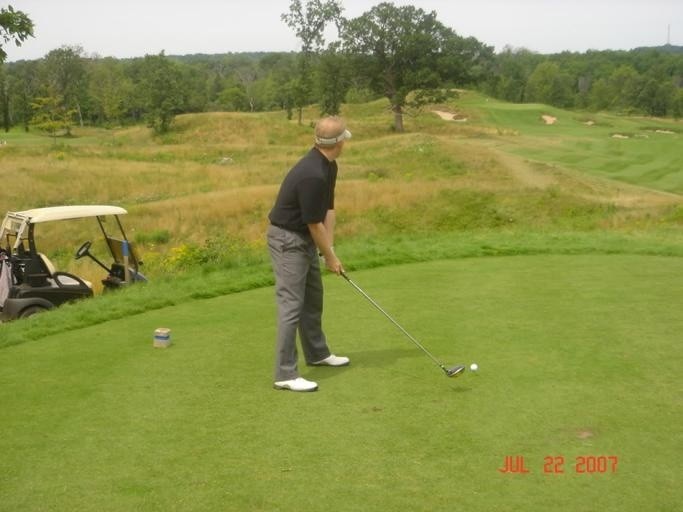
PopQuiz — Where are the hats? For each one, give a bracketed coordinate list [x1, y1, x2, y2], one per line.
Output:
[315, 129, 352, 144]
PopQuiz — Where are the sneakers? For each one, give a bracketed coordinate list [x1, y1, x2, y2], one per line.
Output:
[273, 375, 319, 392]
[308, 353, 350, 367]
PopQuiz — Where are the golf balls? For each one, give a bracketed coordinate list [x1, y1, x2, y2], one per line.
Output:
[470, 364, 476, 370]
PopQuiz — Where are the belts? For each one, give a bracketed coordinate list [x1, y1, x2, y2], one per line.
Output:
[271, 221, 308, 233]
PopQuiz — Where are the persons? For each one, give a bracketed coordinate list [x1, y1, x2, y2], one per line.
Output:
[265, 115, 350, 392]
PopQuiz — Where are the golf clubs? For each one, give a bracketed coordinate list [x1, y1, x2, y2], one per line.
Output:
[318, 251, 463, 376]
